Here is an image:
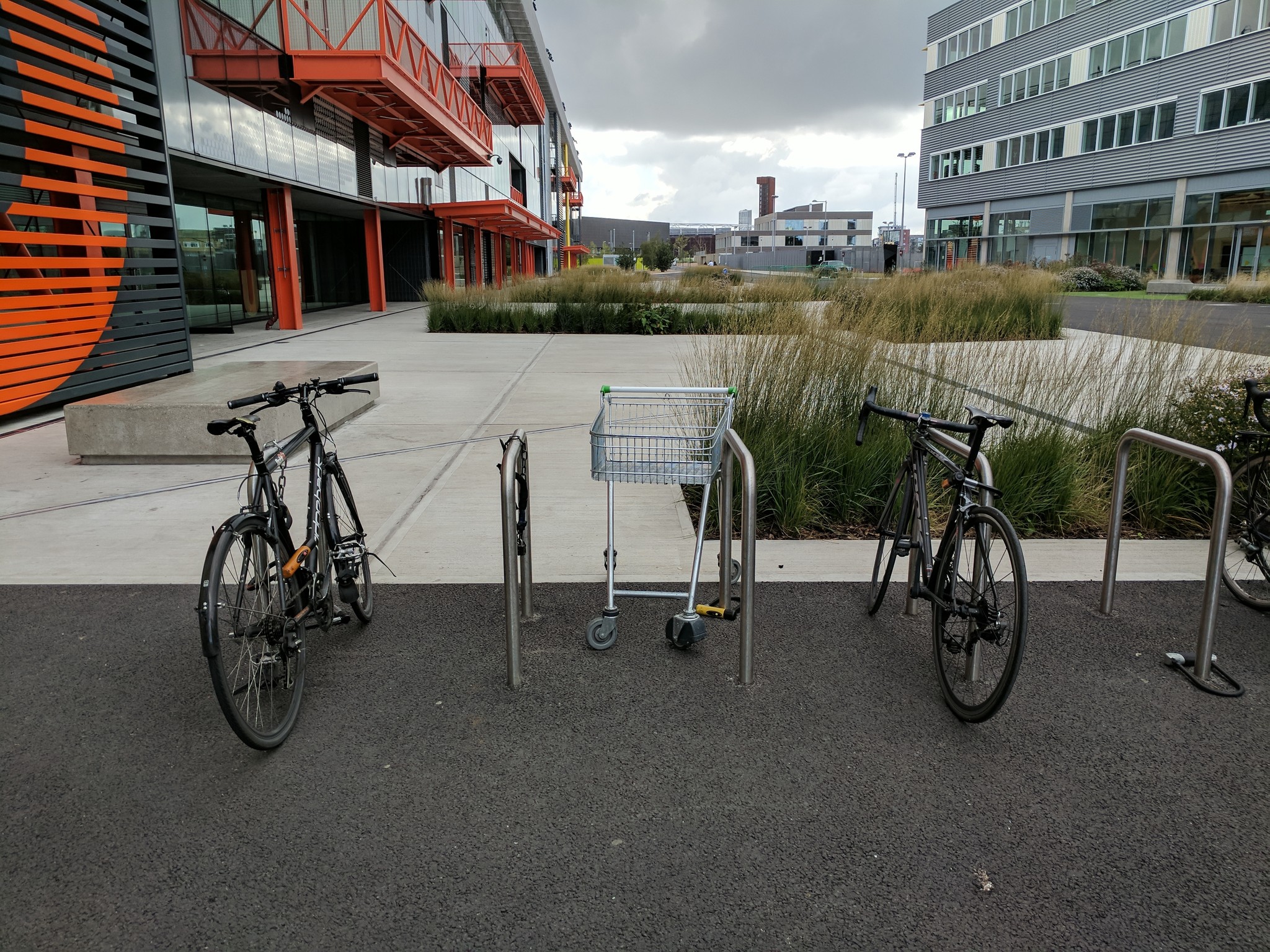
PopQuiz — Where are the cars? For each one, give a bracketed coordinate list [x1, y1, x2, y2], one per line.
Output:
[813, 260, 854, 278]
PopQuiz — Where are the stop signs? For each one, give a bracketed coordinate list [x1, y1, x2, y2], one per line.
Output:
[899, 249, 902, 256]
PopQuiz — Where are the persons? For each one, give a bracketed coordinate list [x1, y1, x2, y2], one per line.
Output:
[707, 260, 714, 266]
[704, 262, 707, 265]
[713, 261, 717, 266]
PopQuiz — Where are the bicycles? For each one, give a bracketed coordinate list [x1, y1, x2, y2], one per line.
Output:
[192, 372, 376, 754]
[854, 386, 1030, 723]
[1218, 377, 1270, 613]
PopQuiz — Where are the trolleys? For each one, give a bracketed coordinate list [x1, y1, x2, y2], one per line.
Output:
[587, 384, 740, 651]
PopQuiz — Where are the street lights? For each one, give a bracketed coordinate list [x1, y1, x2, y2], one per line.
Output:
[718, 229, 727, 266]
[882, 221, 893, 244]
[608, 242, 614, 254]
[703, 243, 706, 251]
[896, 152, 916, 247]
[803, 226, 812, 249]
[765, 214, 777, 266]
[771, 195, 779, 266]
[811, 200, 827, 261]
[739, 222, 749, 270]
